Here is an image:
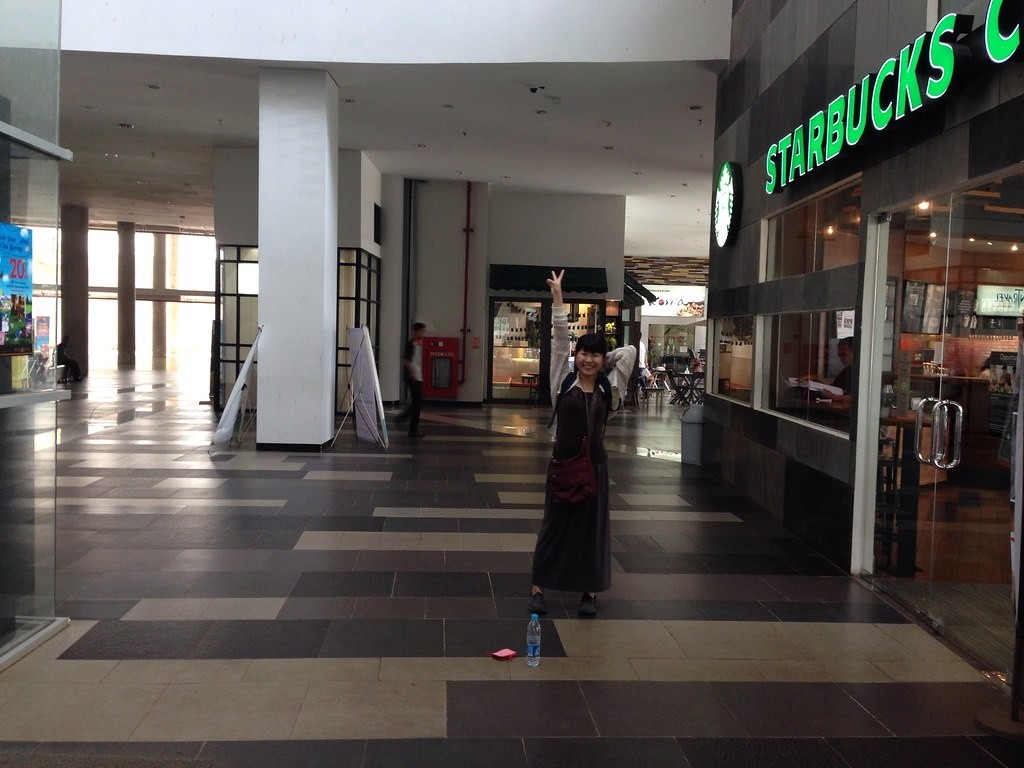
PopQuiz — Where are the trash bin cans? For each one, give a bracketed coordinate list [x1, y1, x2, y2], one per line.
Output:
[679, 404, 705, 466]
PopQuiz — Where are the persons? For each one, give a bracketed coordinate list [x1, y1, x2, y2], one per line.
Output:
[393, 323, 426, 437]
[9, 294, 26, 339]
[638, 332, 654, 400]
[671, 349, 701, 397]
[529, 269, 636, 616]
[979, 363, 1016, 393]
[797, 336, 854, 401]
[52, 336, 84, 381]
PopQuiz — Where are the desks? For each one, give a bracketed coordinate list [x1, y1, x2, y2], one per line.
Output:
[652, 369, 671, 391]
[796, 399, 949, 577]
[673, 373, 693, 386]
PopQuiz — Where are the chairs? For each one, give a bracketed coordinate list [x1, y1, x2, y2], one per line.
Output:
[669, 372, 705, 407]
[526, 373, 552, 408]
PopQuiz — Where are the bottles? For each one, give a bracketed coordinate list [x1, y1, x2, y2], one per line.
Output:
[527, 614, 541, 666]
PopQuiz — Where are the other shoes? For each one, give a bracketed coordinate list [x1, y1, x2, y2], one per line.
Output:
[527, 591, 547, 614]
[408, 431, 426, 437]
[581, 593, 597, 617]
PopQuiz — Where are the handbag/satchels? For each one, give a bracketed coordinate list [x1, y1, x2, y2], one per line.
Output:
[545, 451, 598, 507]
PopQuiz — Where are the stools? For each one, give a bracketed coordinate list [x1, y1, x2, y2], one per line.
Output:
[642, 387, 664, 409]
[874, 438, 902, 565]
[520, 375, 535, 383]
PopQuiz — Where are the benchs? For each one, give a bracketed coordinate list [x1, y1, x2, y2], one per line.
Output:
[45, 365, 66, 382]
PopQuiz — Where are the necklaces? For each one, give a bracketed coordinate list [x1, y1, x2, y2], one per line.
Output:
[582, 389, 590, 435]
